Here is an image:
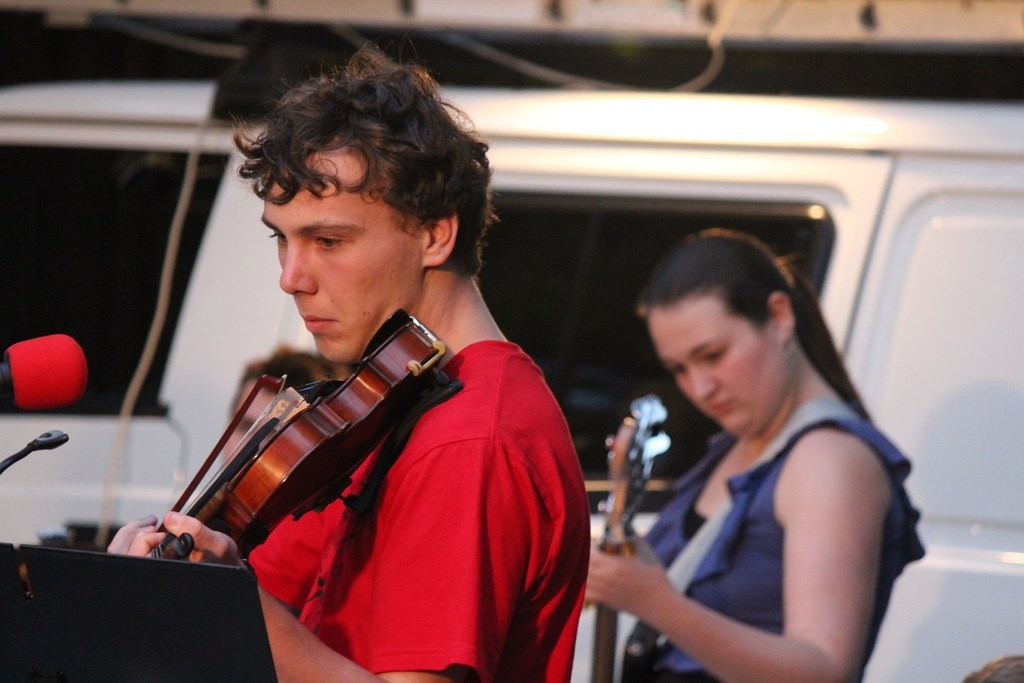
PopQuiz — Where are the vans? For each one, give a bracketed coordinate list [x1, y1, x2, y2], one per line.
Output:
[0, 76, 1024, 683]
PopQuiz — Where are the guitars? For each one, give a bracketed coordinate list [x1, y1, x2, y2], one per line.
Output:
[589, 392, 673, 681]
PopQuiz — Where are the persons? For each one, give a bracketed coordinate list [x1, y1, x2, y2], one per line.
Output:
[584, 229, 924, 681]
[105, 50, 591, 682]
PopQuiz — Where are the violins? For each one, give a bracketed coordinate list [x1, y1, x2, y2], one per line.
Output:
[149, 315, 448, 563]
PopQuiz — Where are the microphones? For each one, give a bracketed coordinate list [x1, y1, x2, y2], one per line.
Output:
[0, 332, 89, 410]
